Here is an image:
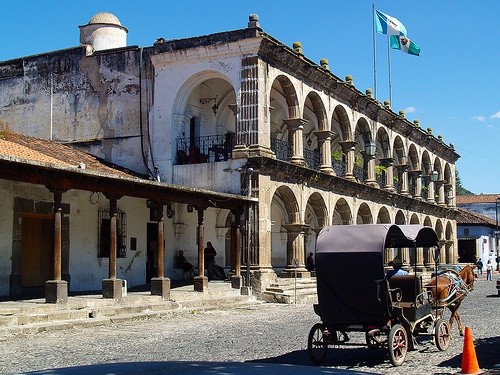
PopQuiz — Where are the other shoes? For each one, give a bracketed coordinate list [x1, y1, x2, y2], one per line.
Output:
[220, 277, 228, 280]
[189, 278, 193, 280]
[194, 273, 199, 276]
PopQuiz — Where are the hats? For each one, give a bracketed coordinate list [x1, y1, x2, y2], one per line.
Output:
[388, 257, 403, 266]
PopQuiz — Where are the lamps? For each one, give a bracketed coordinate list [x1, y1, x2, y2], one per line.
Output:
[200, 95, 219, 116]
[303, 132, 313, 149]
[419, 171, 439, 181]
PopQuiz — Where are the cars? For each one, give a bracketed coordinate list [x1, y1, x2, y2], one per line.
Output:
[496, 277, 500, 297]
[469, 264, 479, 278]
[438, 265, 462, 273]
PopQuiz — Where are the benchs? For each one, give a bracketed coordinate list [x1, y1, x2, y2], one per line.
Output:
[386, 274, 431, 323]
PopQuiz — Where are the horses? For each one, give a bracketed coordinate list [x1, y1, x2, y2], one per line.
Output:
[427, 265, 477, 340]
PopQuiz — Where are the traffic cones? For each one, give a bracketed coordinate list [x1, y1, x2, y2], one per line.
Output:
[454, 325, 486, 375]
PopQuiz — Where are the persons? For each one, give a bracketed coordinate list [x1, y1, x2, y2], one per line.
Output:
[477, 258, 483, 276]
[307, 252, 316, 271]
[387, 257, 430, 301]
[203, 241, 217, 282]
[175, 250, 200, 280]
[486, 260, 493, 281]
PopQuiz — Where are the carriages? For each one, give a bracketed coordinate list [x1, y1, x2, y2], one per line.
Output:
[308, 223, 478, 366]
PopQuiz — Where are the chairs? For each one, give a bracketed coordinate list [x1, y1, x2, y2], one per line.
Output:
[173, 256, 191, 280]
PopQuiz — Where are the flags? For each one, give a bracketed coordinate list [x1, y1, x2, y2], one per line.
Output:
[375, 7, 408, 37]
[390, 35, 422, 56]
